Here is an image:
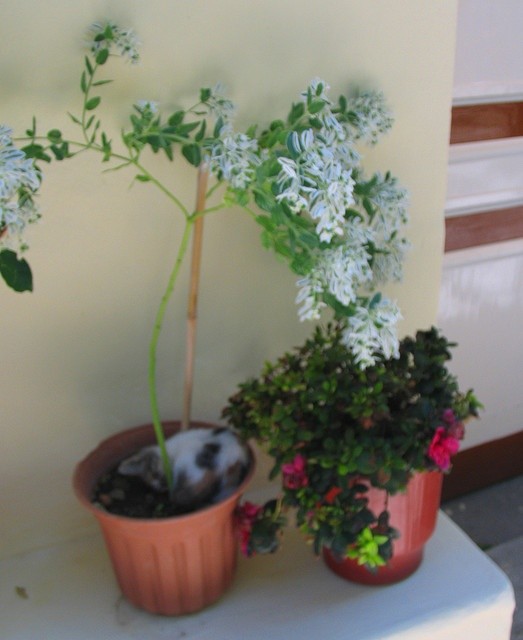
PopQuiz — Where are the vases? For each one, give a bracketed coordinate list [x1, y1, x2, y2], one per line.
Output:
[301, 458, 444, 587]
[69, 418, 257, 618]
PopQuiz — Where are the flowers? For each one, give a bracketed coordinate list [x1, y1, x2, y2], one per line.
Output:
[0, 12, 414, 496]
[221, 320, 486, 576]
